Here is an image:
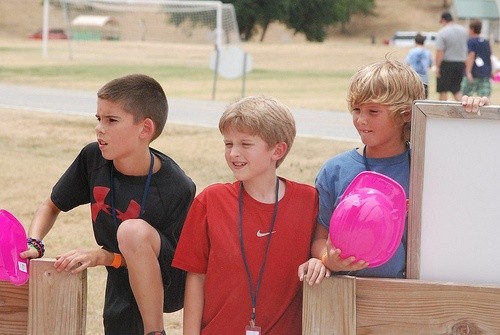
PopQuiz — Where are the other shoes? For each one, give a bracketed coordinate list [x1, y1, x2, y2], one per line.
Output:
[146, 330, 166, 335]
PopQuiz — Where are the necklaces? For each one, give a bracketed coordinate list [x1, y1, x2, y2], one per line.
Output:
[102, 146, 155, 237]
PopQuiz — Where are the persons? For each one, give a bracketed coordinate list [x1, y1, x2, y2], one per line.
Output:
[171, 93, 330, 334]
[20, 73, 196, 335]
[404, 11, 498, 106]
[312, 52, 490, 280]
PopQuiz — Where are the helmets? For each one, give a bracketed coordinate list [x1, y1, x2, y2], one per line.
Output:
[0, 209, 29, 286]
[330, 171, 406, 268]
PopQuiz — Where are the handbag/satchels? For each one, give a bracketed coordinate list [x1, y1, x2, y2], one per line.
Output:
[490, 55, 500, 81]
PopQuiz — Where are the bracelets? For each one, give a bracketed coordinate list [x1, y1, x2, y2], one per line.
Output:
[110, 253, 122, 268]
[25, 237, 45, 258]
